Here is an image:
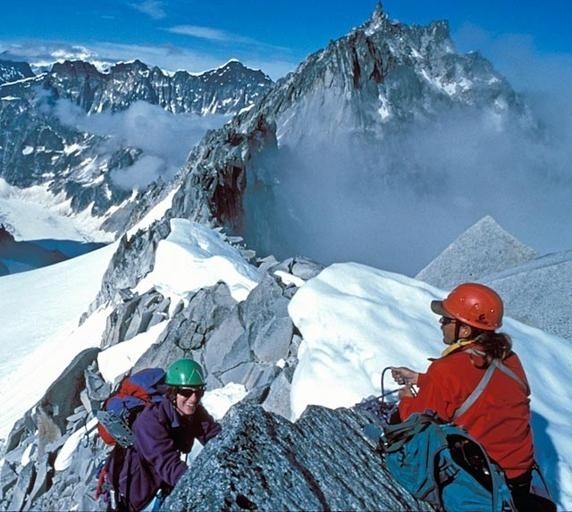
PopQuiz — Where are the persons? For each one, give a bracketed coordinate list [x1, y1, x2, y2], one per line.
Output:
[94, 357, 222, 511]
[388, 283, 558, 511]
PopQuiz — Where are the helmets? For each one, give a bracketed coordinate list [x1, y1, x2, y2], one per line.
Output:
[441, 282, 504, 331]
[165, 359, 207, 387]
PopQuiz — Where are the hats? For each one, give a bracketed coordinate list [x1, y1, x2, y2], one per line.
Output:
[431, 301, 455, 319]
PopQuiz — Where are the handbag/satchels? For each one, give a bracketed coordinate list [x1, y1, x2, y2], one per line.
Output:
[380, 407, 518, 512]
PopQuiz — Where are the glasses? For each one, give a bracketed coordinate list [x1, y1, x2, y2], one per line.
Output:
[178, 389, 204, 397]
[440, 316, 456, 324]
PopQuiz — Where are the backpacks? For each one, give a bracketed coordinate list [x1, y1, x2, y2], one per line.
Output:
[98, 367, 166, 445]
